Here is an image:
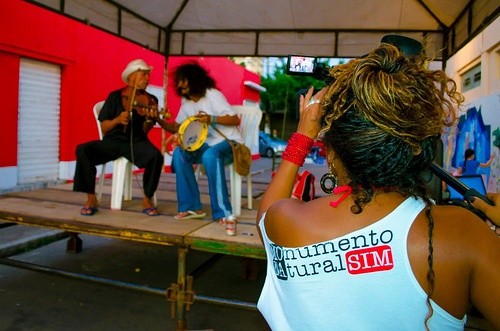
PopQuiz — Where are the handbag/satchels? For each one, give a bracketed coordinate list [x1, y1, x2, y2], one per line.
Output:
[229, 140, 250, 176]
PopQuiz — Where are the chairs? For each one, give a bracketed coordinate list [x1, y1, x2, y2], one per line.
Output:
[194, 105, 262, 218]
[94, 101, 158, 211]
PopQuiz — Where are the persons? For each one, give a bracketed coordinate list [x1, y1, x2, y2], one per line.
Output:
[256, 43, 500, 331]
[143, 63, 244, 224]
[457, 149, 494, 175]
[73, 59, 164, 216]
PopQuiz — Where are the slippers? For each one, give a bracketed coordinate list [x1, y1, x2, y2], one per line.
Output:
[79, 207, 98, 216]
[174, 211, 206, 220]
[142, 207, 160, 216]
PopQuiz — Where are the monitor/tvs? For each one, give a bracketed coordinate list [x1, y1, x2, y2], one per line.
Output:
[446, 175, 487, 202]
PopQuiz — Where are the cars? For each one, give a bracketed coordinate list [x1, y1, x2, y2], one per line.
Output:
[258, 131, 289, 159]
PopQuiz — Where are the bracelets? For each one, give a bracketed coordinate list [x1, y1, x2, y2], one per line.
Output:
[283, 132, 314, 167]
[210, 114, 217, 125]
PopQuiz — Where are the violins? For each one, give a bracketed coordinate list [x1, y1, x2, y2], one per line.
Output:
[122, 87, 172, 118]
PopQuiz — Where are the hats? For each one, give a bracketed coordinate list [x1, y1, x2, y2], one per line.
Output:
[121, 58, 153, 83]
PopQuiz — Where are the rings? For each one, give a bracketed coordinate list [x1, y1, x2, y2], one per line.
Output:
[305, 95, 315, 108]
[314, 100, 320, 103]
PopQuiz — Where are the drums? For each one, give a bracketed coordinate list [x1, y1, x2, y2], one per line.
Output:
[177, 116, 209, 152]
